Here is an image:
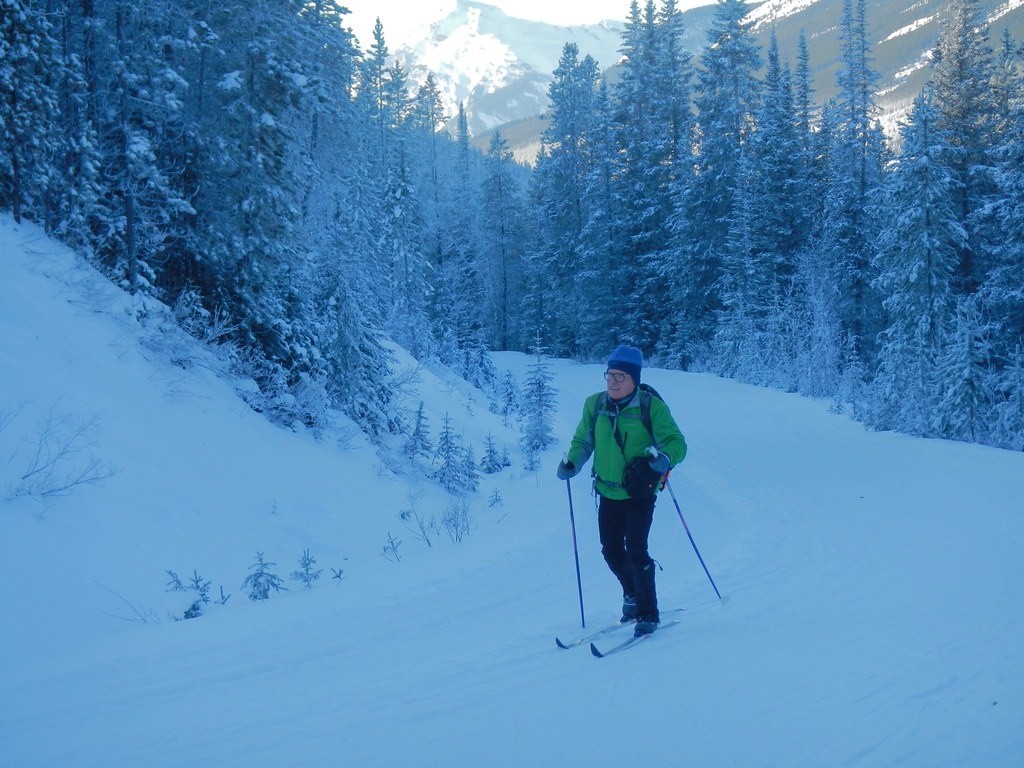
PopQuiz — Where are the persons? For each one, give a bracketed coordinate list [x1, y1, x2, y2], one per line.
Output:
[557, 345, 688, 633]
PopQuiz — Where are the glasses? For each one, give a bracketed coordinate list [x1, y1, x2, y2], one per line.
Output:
[604, 372, 630, 382]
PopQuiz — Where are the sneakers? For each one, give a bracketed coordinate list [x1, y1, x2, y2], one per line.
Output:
[632, 618, 660, 633]
[623, 595, 638, 615]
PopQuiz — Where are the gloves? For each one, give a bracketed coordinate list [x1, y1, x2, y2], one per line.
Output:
[557, 461, 576, 480]
[649, 454, 669, 474]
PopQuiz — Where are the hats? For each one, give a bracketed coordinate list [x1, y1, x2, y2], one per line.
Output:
[607, 345, 643, 384]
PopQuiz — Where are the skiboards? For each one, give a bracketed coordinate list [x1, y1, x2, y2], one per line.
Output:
[555, 606, 687, 658]
